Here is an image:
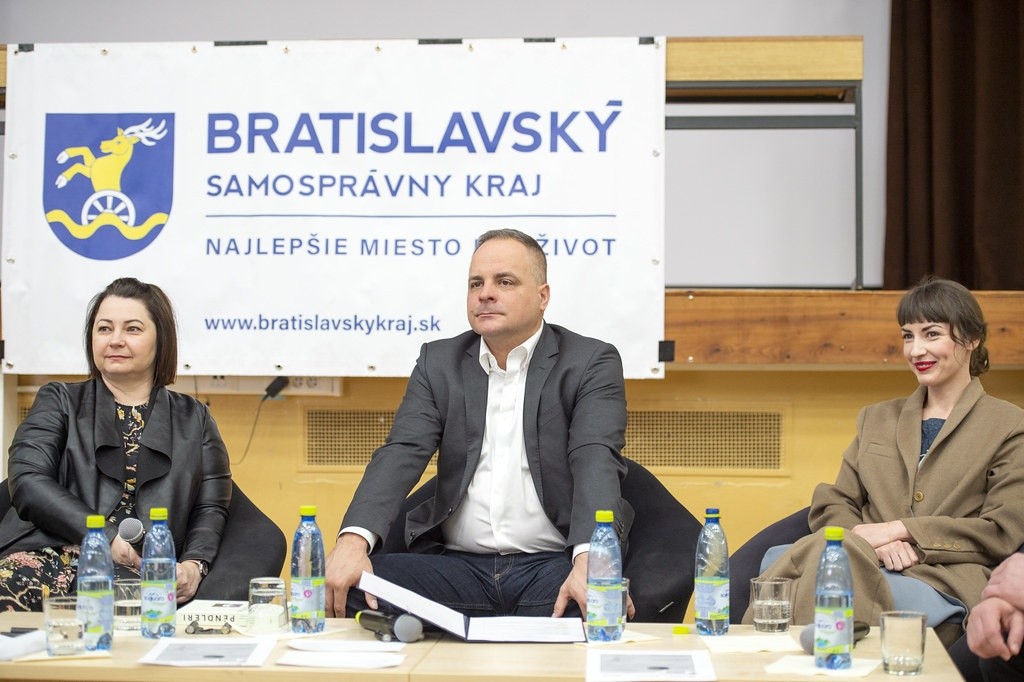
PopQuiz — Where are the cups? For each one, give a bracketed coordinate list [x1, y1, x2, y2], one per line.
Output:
[880, 611, 928, 676]
[248, 577, 288, 632]
[42, 596, 78, 656]
[113, 578, 141, 631]
[750, 577, 791, 632]
[622, 577, 630, 630]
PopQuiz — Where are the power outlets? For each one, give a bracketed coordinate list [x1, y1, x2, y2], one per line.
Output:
[239, 376, 344, 398]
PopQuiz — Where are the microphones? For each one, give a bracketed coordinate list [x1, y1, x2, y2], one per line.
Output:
[355, 609, 422, 642]
[118, 517, 157, 559]
[800, 620, 870, 654]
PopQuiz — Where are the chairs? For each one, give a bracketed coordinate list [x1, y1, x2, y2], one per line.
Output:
[0, 477, 288, 604]
[343, 455, 710, 621]
[715, 506, 984, 682]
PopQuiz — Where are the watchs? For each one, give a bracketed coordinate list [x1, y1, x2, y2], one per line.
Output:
[191, 559, 209, 576]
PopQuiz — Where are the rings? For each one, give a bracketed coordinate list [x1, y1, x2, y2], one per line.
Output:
[131, 563, 134, 566]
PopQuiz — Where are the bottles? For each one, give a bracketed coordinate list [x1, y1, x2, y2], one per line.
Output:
[77, 514, 113, 650]
[813, 527, 854, 669]
[586, 510, 623, 642]
[140, 507, 176, 639]
[694, 509, 730, 636]
[290, 505, 325, 633]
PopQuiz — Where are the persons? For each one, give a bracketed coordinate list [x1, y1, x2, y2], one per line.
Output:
[946, 542, 1024, 682]
[323, 229, 636, 625]
[740, 280, 1024, 648]
[0, 276, 233, 612]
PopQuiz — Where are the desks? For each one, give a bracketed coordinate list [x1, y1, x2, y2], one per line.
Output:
[0, 610, 966, 682]
